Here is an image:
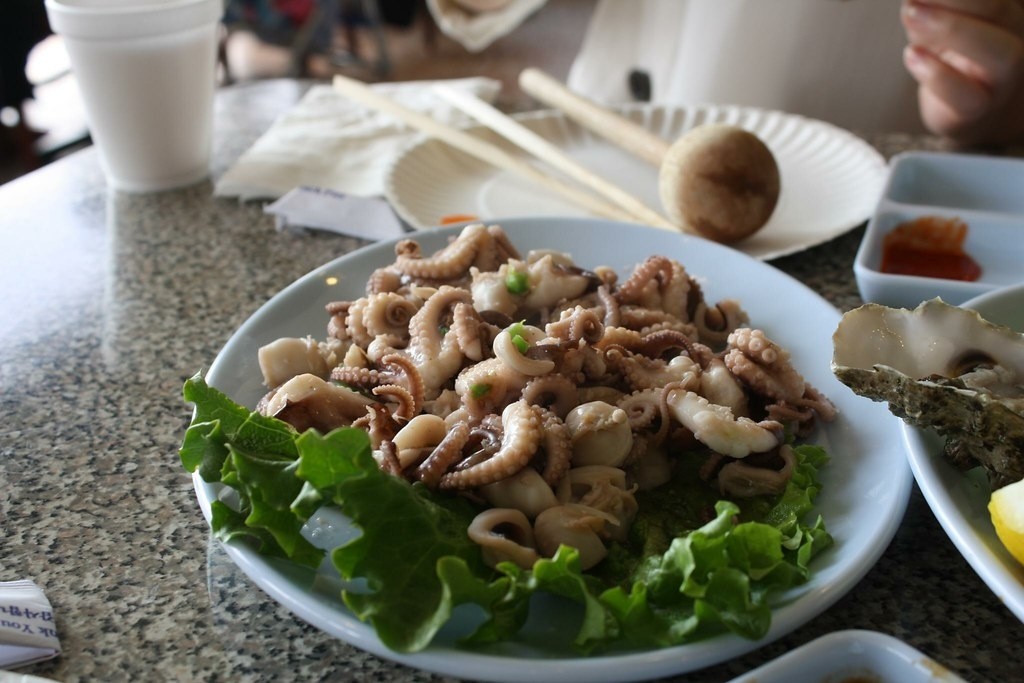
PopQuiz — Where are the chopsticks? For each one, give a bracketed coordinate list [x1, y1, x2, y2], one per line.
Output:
[335, 88, 687, 241]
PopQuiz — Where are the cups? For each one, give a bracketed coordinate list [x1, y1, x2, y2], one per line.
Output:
[46, 0, 227, 194]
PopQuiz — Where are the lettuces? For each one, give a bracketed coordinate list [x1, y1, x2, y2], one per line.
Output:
[177, 368, 833, 655]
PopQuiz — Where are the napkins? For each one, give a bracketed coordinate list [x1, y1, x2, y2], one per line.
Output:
[213, 76, 502, 196]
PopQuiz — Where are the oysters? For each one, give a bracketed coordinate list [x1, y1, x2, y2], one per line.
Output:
[833, 295, 1024, 490]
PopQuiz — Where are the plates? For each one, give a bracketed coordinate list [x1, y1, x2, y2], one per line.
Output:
[189, 217, 912, 683]
[729, 630, 967, 683]
[385, 104, 887, 260]
[853, 150, 1024, 312]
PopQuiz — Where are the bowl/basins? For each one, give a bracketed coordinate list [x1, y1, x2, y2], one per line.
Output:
[903, 286, 1024, 622]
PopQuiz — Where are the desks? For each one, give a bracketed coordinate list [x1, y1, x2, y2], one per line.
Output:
[0, 76, 1024, 683]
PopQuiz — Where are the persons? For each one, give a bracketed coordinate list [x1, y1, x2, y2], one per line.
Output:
[425, 0, 1024, 148]
